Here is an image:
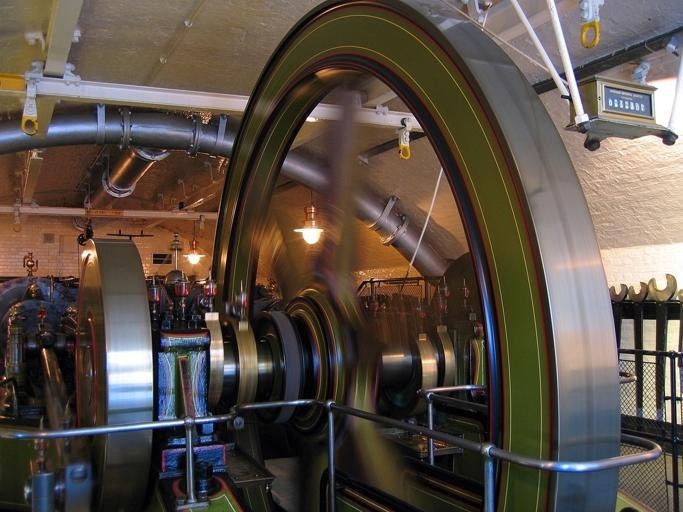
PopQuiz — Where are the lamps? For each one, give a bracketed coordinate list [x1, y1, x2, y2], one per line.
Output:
[292, 186, 324, 245]
[182, 220, 206, 264]
